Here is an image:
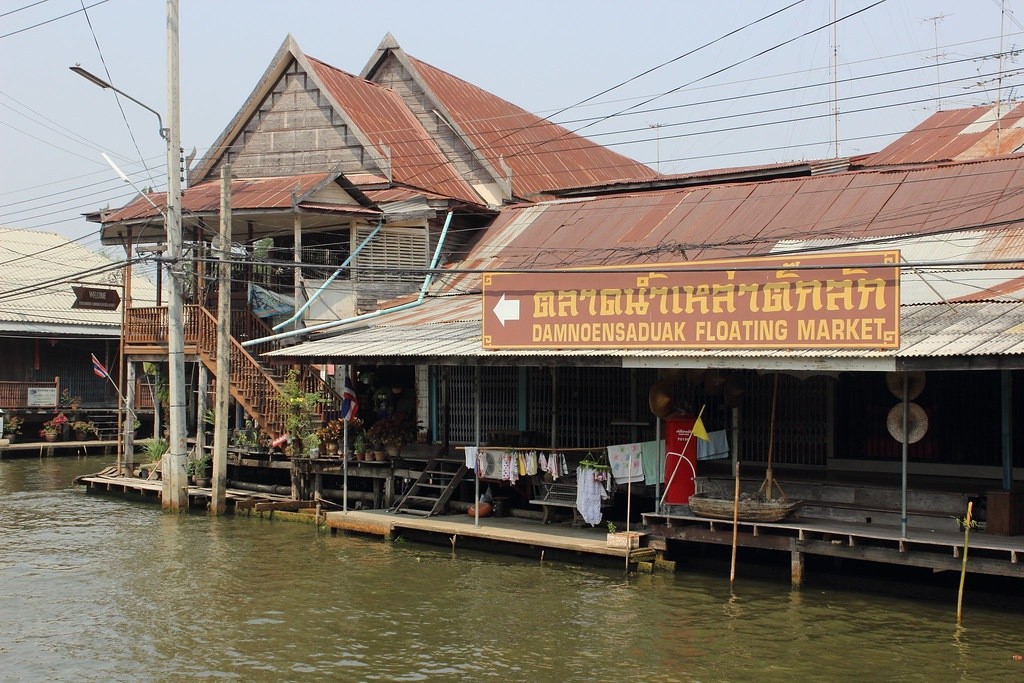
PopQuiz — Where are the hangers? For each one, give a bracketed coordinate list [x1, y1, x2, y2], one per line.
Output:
[504, 446, 564, 454]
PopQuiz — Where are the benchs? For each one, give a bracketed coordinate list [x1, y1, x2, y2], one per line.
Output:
[528, 482, 602, 529]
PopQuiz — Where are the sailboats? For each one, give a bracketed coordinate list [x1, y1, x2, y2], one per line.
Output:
[688, 372, 806, 523]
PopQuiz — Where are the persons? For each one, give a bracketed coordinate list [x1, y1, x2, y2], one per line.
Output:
[652, 384, 693, 421]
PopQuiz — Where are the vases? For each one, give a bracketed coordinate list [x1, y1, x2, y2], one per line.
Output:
[45, 434, 58, 442]
[75, 431, 88, 441]
[61, 405, 69, 410]
[4, 433, 16, 444]
[71, 404, 78, 410]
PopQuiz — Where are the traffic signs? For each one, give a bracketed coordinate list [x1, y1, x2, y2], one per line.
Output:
[71, 285, 121, 310]
[480, 248, 903, 352]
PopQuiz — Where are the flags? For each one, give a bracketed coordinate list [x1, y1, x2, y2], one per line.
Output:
[342, 377, 358, 422]
[92, 356, 108, 377]
[144, 362, 156, 376]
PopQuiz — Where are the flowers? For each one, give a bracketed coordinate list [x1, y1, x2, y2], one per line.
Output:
[69, 421, 98, 435]
[40, 413, 68, 437]
[61, 389, 72, 406]
[4, 417, 18, 433]
[70, 395, 81, 404]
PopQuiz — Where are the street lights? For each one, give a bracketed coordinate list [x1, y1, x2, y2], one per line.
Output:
[70, 65, 190, 512]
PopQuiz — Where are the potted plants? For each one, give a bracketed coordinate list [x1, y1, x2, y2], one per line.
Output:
[236, 435, 263, 452]
[142, 467, 158, 479]
[190, 454, 210, 488]
[141, 436, 168, 470]
[308, 418, 406, 461]
[606, 520, 649, 549]
